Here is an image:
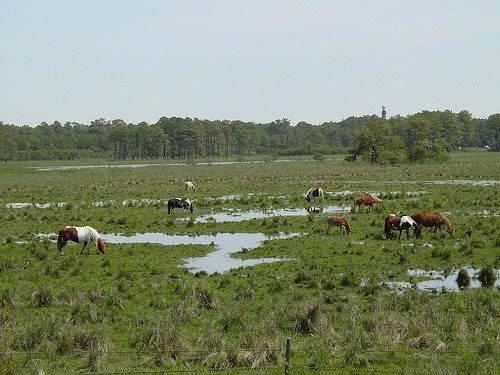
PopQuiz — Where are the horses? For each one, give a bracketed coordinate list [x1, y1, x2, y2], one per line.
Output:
[185, 182, 196, 193]
[326, 216, 351, 235]
[57, 225, 106, 255]
[168, 197, 194, 214]
[305, 187, 324, 203]
[383, 214, 423, 240]
[353, 195, 385, 212]
[410, 211, 454, 237]
